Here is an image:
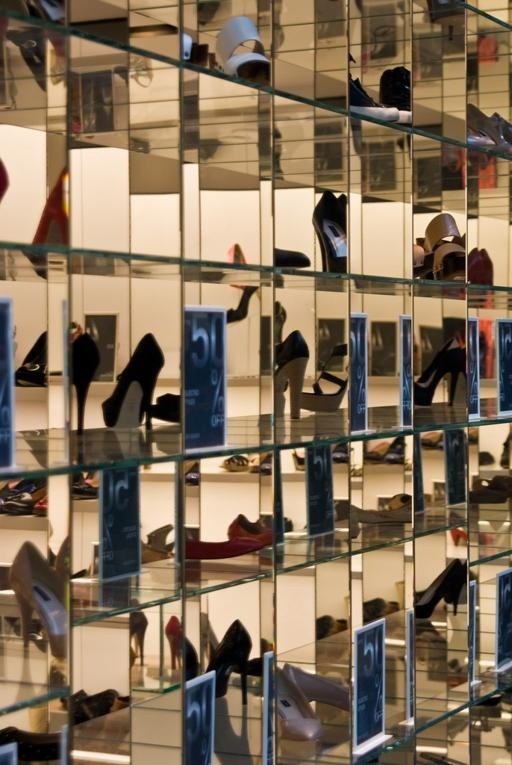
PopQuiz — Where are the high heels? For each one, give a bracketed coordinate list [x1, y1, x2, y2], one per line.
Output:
[166, 616, 185, 670]
[276, 333, 310, 421]
[310, 193, 348, 274]
[153, 394, 179, 421]
[69, 333, 101, 436]
[414, 559, 462, 618]
[103, 333, 164, 430]
[413, 339, 464, 407]
[8, 543, 69, 658]
[226, 286, 260, 323]
[202, 620, 252, 704]
[302, 345, 348, 414]
[185, 636, 198, 681]
[130, 612, 147, 667]
[275, 249, 308, 265]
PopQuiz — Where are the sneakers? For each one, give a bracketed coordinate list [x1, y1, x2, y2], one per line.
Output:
[381, 65, 416, 124]
[349, 70, 402, 122]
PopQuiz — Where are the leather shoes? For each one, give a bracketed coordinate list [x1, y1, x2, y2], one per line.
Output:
[14, 330, 45, 385]
[481, 475, 511, 499]
[480, 489, 504, 506]
[350, 517, 361, 538]
[0, 473, 99, 517]
[291, 427, 478, 468]
[468, 100, 512, 155]
[229, 515, 273, 546]
[351, 501, 412, 524]
[184, 537, 264, 559]
[227, 245, 248, 290]
[284, 664, 348, 711]
[276, 669, 321, 742]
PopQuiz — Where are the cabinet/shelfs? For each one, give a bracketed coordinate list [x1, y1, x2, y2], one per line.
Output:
[1, 0, 512, 764]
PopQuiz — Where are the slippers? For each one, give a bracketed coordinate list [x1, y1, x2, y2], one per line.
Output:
[414, 246, 433, 282]
[415, 214, 465, 283]
[181, 15, 269, 83]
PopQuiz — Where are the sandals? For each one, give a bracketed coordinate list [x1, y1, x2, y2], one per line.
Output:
[222, 455, 274, 474]
